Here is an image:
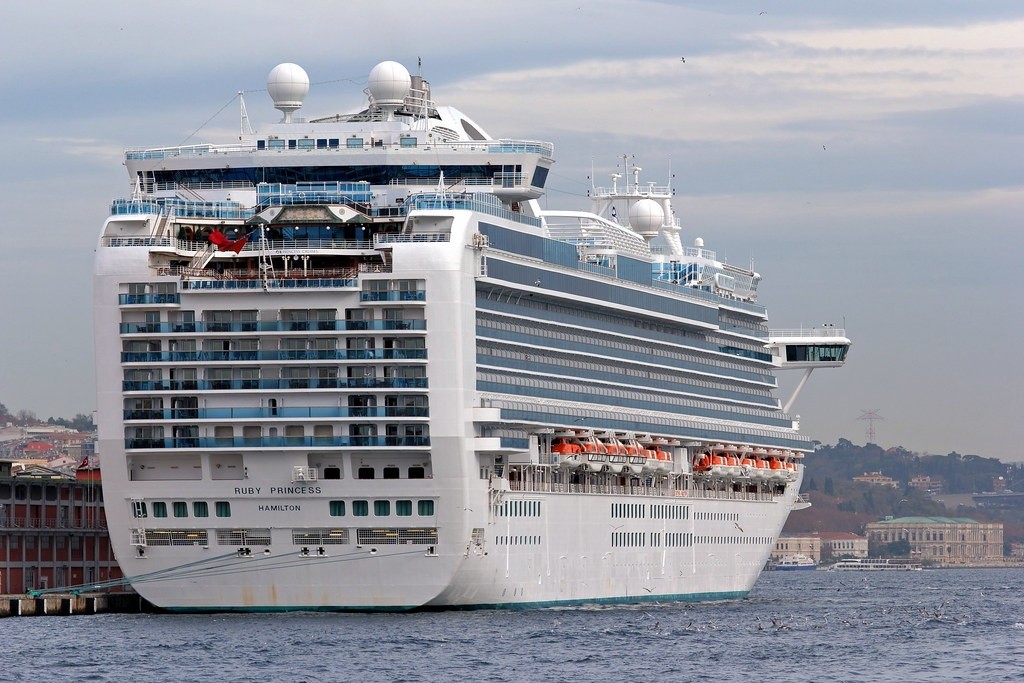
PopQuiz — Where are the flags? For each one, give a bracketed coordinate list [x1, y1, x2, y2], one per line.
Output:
[612, 205, 619, 224]
[208, 227, 259, 254]
[78, 456, 88, 468]
[89, 459, 93, 469]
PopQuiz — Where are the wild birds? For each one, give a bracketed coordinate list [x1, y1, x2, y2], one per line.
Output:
[759, 11, 768, 16]
[680, 56, 686, 63]
[533, 569, 1024, 675]
[823, 145, 827, 151]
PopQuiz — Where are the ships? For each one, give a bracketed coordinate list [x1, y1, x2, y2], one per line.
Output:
[94, 55, 853, 614]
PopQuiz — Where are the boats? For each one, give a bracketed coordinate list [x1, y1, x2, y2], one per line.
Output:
[550, 429, 674, 475]
[825, 559, 924, 571]
[695, 451, 799, 484]
[773, 552, 818, 570]
